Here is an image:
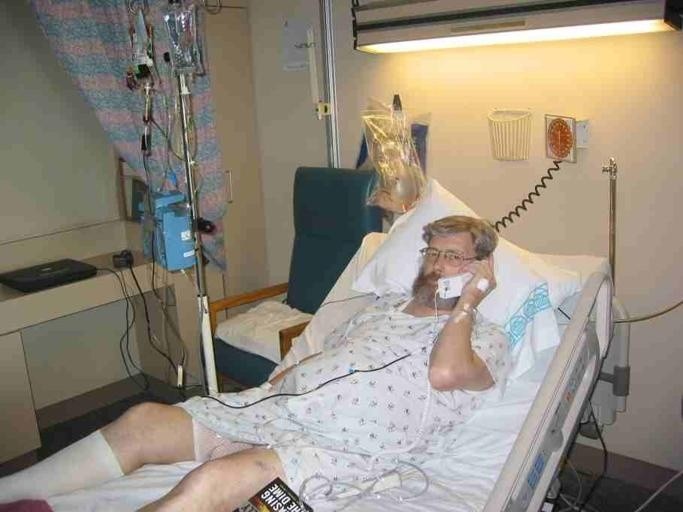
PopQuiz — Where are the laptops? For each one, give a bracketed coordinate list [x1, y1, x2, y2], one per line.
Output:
[0, 258, 97, 293]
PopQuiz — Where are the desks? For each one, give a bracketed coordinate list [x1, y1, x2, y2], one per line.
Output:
[0, 250, 227, 466]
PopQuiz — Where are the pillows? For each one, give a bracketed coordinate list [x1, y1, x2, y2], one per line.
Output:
[349, 178, 584, 388]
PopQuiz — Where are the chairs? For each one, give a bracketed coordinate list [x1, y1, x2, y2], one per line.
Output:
[199, 165, 384, 395]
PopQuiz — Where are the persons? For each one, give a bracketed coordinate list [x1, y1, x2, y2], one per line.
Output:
[0, 214, 514, 512]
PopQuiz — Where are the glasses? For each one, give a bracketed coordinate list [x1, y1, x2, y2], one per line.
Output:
[419, 247, 476, 267]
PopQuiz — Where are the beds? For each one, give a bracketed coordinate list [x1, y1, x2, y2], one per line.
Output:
[1, 230, 614, 512]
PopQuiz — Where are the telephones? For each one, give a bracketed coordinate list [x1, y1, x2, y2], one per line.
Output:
[437, 272, 474, 300]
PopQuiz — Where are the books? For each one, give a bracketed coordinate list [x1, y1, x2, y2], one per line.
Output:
[231, 476, 313, 511]
